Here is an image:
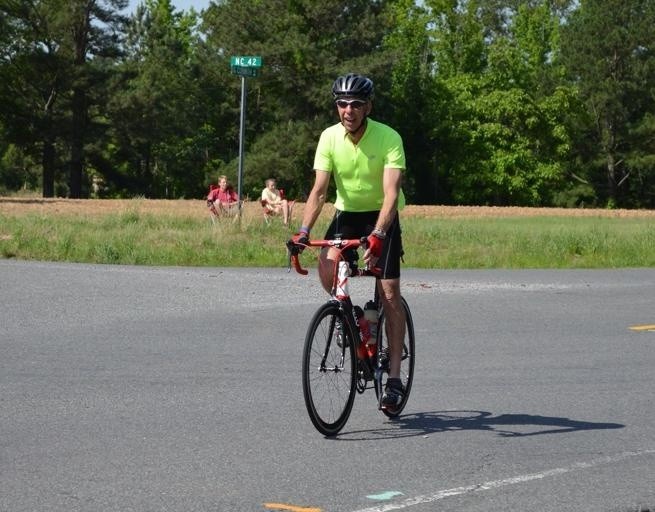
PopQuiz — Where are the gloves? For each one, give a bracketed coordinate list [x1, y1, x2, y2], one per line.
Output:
[363, 227, 387, 259]
[285, 229, 309, 256]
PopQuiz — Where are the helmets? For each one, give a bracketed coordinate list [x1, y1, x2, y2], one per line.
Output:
[330, 72, 376, 102]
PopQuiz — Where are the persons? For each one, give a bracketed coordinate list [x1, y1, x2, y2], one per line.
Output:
[204, 175, 244, 219]
[261, 177, 291, 229]
[283, 67, 409, 414]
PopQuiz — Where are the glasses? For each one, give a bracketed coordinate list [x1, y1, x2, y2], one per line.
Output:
[334, 98, 370, 109]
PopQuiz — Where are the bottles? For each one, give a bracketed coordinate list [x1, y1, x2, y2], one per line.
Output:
[354, 300, 378, 344]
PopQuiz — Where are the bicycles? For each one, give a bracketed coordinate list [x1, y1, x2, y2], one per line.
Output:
[285, 237, 415, 436]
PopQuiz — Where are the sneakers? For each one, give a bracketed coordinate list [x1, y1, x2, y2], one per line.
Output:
[335, 317, 350, 348]
[379, 377, 407, 408]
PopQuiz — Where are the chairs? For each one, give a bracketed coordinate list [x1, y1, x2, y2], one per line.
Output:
[259, 189, 298, 230]
[207, 184, 244, 233]
